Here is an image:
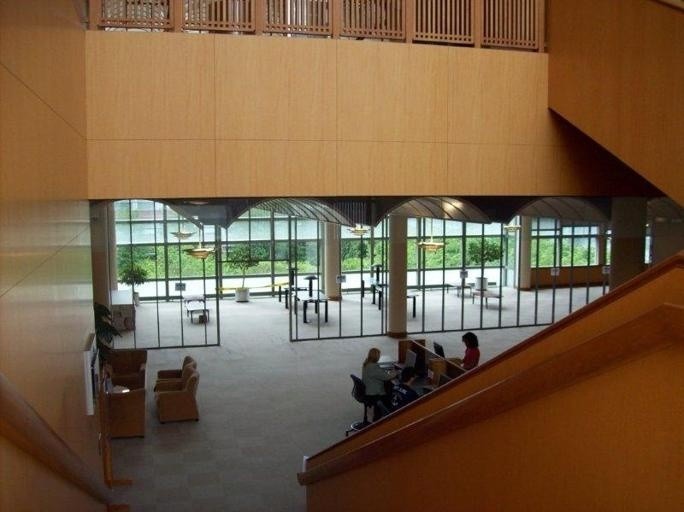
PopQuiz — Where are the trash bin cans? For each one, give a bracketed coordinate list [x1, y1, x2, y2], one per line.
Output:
[236, 288, 249, 302]
[476, 277, 488, 291]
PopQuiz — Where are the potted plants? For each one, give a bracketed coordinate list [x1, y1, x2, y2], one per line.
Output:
[119, 245, 148, 307]
[468, 239, 504, 290]
[229, 246, 259, 302]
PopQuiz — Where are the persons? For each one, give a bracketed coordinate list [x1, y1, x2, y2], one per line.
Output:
[462, 332, 481, 370]
[360, 348, 419, 422]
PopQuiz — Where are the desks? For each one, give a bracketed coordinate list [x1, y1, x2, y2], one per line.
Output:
[182, 293, 209, 323]
[472, 290, 504, 309]
[284, 287, 328, 323]
[371, 284, 419, 318]
[447, 285, 472, 297]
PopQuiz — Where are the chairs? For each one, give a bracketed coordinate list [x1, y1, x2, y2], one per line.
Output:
[345, 374, 388, 437]
[103, 349, 200, 438]
[374, 401, 389, 423]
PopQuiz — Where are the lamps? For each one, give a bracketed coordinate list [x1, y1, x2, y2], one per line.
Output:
[503, 221, 520, 233]
[415, 218, 444, 251]
[346, 223, 368, 236]
[170, 216, 215, 259]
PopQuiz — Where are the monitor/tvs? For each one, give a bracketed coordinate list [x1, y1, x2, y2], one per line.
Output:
[405, 349, 417, 368]
[438, 374, 452, 388]
[434, 342, 445, 358]
